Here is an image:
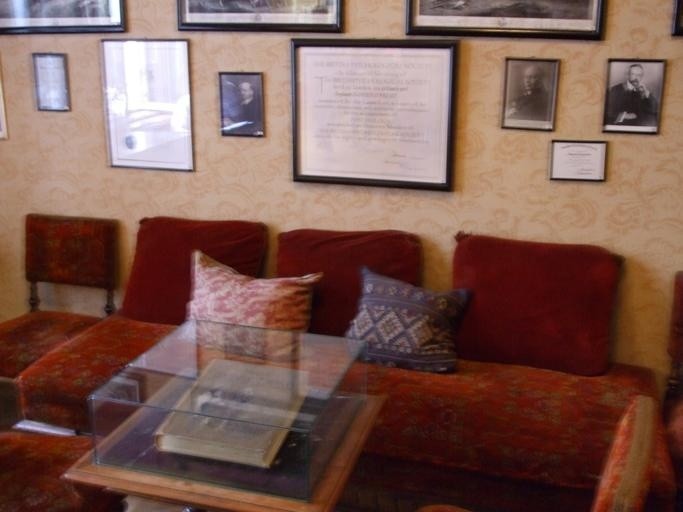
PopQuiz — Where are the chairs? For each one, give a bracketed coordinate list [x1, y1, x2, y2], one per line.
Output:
[658, 265, 683, 469]
[414, 390, 659, 512]
[1, 209, 120, 393]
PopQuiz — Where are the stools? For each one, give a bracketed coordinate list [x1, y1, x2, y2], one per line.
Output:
[0, 428, 132, 511]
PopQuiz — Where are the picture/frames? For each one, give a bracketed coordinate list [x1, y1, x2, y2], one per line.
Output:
[0, 55, 12, 142]
[287, 35, 457, 194]
[29, 50, 72, 115]
[600, 56, 667, 138]
[667, 0, 681, 39]
[546, 138, 609, 184]
[173, 1, 347, 36]
[500, 55, 563, 134]
[97, 37, 196, 176]
[0, 0, 128, 38]
[215, 69, 266, 140]
[402, 1, 608, 45]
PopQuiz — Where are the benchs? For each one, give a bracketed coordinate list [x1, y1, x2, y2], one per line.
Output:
[11, 312, 679, 505]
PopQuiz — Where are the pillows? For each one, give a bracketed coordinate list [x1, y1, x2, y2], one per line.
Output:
[116, 212, 270, 329]
[272, 223, 427, 335]
[179, 249, 323, 340]
[444, 231, 629, 377]
[339, 261, 481, 372]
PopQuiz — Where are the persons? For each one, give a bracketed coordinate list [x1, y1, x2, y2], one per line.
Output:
[603, 64, 658, 126]
[505, 65, 549, 121]
[224, 83, 262, 136]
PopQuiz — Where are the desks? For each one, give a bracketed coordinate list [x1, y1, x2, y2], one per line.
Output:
[52, 314, 393, 511]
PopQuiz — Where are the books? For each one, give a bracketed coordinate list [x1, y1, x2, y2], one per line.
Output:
[150, 358, 309, 468]
